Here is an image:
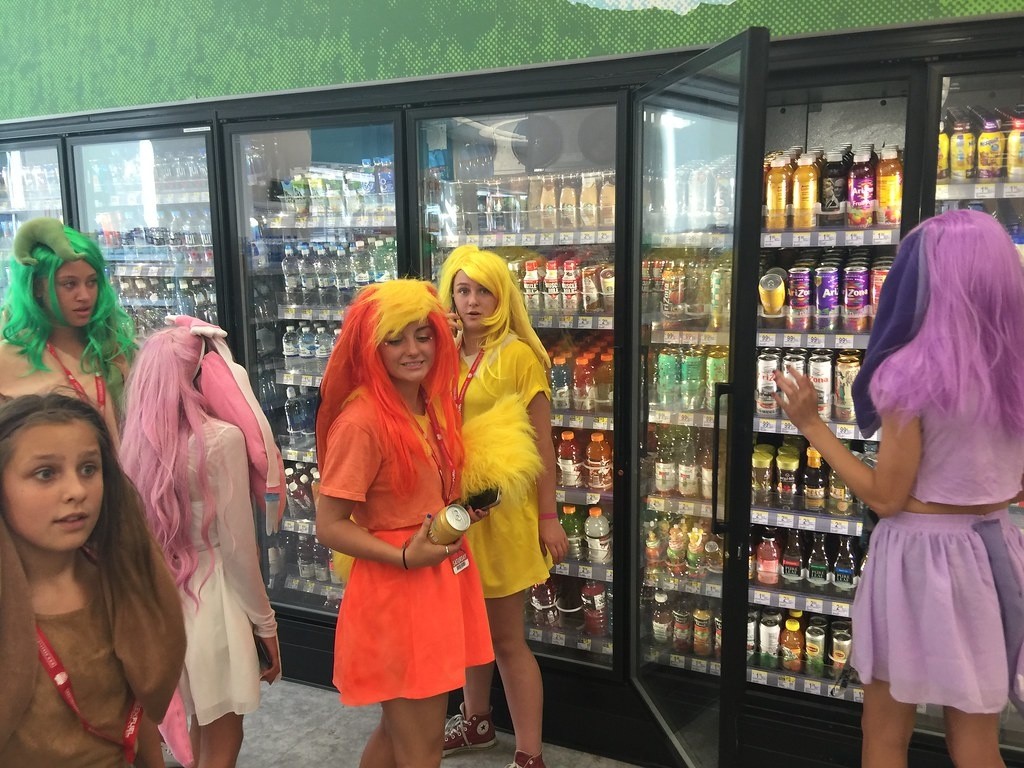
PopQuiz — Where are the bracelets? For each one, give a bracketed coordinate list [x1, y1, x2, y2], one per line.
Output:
[538, 513, 558, 520]
[402, 548, 408, 570]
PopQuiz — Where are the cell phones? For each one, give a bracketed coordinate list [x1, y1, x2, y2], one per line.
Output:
[463, 486, 502, 513]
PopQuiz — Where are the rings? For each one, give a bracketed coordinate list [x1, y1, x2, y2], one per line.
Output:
[446, 545, 450, 554]
[486, 512, 488, 516]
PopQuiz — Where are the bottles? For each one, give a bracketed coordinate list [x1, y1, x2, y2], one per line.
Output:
[83, 143, 397, 611]
[780, 619, 805, 674]
[435, 166, 614, 639]
[638, 423, 723, 660]
[747, 434, 878, 596]
[938, 119, 1024, 245]
[763, 141, 904, 229]
[786, 609, 806, 633]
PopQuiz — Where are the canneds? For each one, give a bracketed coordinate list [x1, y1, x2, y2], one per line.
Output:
[656, 155, 735, 232]
[582, 264, 614, 316]
[756, 249, 894, 423]
[400, 504, 470, 549]
[803, 614, 853, 681]
[746, 606, 787, 669]
[649, 260, 730, 414]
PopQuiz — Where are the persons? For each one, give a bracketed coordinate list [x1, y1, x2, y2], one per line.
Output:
[315, 279, 490, 768]
[0, 218, 141, 458]
[770, 209, 1023, 768]
[437, 245, 571, 768]
[0, 392, 188, 768]
[118, 314, 288, 767]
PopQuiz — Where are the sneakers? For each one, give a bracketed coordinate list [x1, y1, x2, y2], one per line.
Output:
[442, 702, 496, 758]
[505, 747, 546, 768]
